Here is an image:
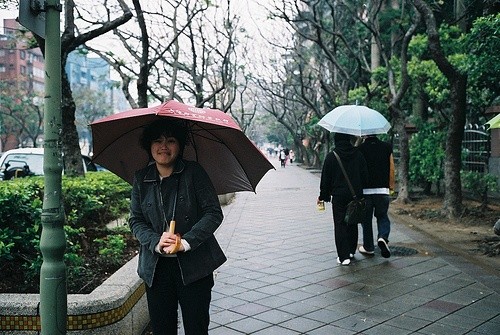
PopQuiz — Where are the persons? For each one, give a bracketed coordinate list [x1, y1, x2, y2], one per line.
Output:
[357, 134, 395, 259]
[317, 133, 369, 265]
[267, 146, 295, 168]
[128, 122, 227, 335]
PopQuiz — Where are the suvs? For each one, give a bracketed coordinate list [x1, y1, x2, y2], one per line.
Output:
[0, 148, 97, 181]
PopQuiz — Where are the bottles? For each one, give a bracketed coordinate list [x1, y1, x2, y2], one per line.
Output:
[317, 200, 325, 212]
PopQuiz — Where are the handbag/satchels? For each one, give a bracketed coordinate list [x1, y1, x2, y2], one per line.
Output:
[344, 197, 372, 224]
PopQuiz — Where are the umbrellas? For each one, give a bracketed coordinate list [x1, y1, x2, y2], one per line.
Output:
[87, 100, 276, 253]
[484, 113, 500, 131]
[317, 100, 391, 137]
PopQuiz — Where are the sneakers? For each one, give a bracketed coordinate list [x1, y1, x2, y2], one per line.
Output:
[337, 252, 353, 265]
[359, 246, 374, 253]
[378, 237, 390, 258]
